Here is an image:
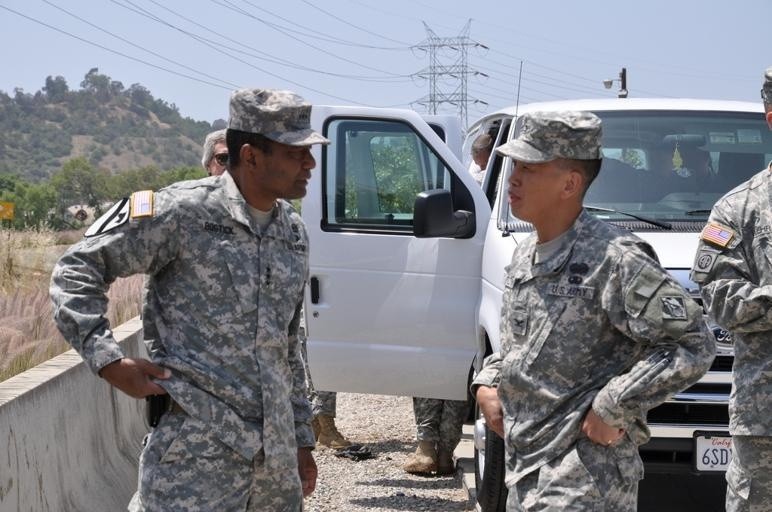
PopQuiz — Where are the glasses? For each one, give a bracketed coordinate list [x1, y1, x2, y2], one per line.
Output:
[213, 153, 228, 166]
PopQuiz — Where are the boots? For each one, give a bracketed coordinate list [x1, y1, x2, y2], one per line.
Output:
[312, 413, 351, 448]
[403, 439, 455, 475]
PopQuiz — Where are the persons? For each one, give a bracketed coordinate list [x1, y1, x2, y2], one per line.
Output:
[401, 391, 475, 476]
[469, 112, 717, 512]
[689, 67, 771, 510]
[201, 126, 232, 175]
[297, 305, 350, 450]
[49, 87, 331, 511]
[468, 125, 733, 206]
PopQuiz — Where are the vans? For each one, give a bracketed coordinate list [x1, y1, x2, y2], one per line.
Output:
[300, 66, 768, 512]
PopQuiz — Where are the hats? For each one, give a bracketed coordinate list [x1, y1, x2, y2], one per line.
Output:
[228, 88, 331, 147]
[495, 110, 606, 163]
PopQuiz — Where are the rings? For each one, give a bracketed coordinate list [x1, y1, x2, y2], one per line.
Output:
[606, 439, 612, 446]
